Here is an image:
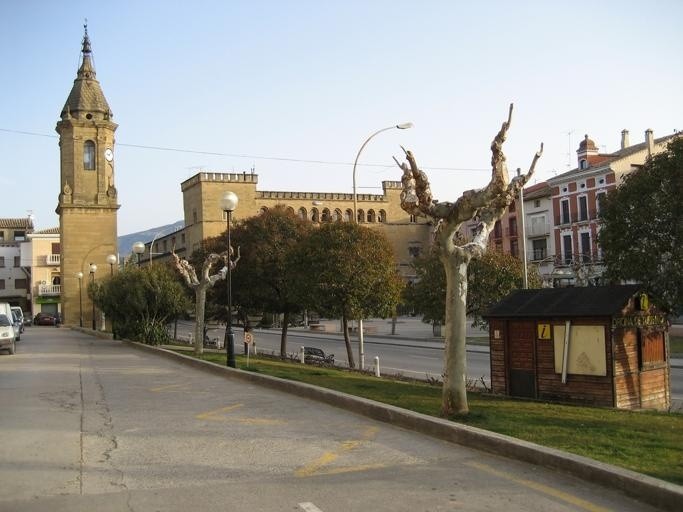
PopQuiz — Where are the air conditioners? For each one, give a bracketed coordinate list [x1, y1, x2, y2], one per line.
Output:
[39, 279, 46, 285]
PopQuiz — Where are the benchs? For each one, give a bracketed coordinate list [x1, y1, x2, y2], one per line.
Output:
[302, 346, 335, 366]
[347, 325, 376, 334]
[309, 323, 338, 333]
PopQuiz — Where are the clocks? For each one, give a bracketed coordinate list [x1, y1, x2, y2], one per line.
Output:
[104, 148, 114, 162]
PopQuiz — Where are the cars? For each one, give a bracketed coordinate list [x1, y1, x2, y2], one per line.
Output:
[296, 345, 334, 366]
[0, 302, 31, 355]
[33, 312, 56, 326]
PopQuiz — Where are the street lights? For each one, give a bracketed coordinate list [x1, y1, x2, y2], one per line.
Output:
[131, 241, 145, 269]
[88, 264, 97, 330]
[80, 242, 113, 327]
[348, 120, 413, 332]
[148, 225, 182, 269]
[106, 254, 116, 276]
[217, 190, 243, 368]
[76, 272, 83, 327]
